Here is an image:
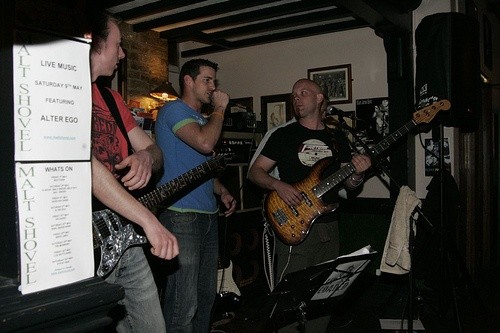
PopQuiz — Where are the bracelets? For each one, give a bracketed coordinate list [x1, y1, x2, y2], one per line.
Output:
[271, 176, 279, 189]
[212, 110, 223, 118]
[351, 176, 364, 184]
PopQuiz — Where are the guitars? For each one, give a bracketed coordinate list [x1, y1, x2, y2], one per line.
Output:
[92, 147, 235, 276]
[264, 99, 451, 246]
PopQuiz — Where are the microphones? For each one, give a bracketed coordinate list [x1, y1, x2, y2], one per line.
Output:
[326, 105, 357, 121]
[227, 101, 248, 110]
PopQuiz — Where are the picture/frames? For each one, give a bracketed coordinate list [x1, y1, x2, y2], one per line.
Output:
[307, 64, 352, 104]
[465, 0, 478, 21]
[260, 94, 293, 137]
[483, 15, 492, 72]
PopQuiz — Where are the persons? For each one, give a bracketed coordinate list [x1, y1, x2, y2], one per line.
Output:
[90, 9, 181, 333]
[248, 79, 371, 333]
[155, 57, 238, 332]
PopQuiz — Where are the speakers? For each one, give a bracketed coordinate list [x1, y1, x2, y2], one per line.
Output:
[415, 11, 480, 135]
[218, 208, 271, 290]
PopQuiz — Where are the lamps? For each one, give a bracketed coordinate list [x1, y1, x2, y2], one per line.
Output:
[148, 81, 178, 115]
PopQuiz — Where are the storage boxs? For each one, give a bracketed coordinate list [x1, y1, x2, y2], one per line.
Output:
[217, 163, 266, 217]
